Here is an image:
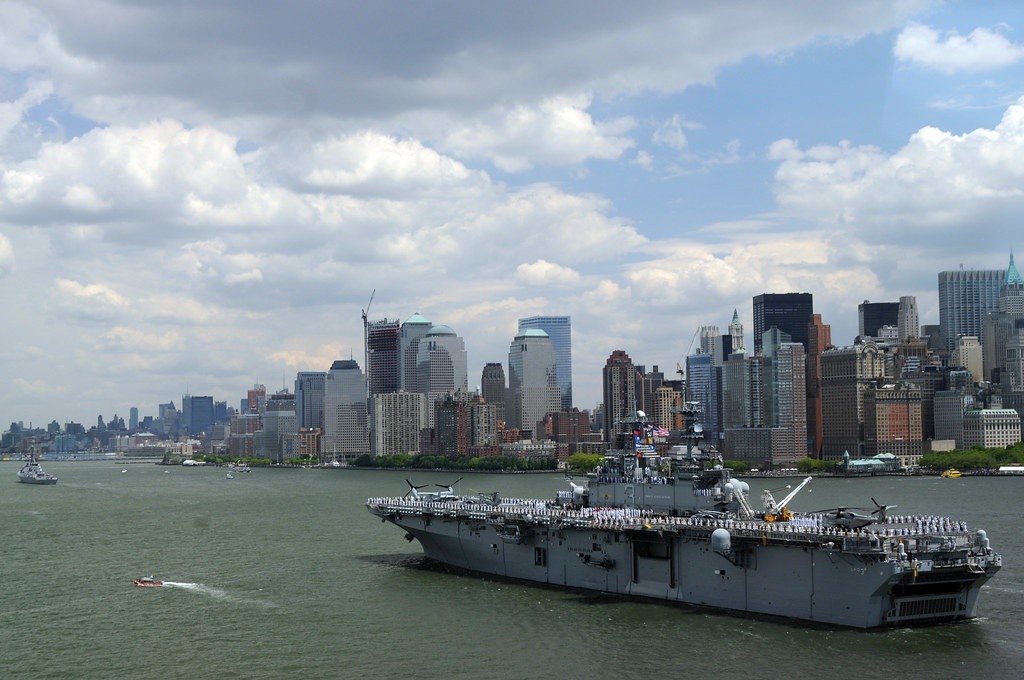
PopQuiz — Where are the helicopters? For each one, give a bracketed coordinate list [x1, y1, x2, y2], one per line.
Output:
[806, 497, 898, 530]
[403, 477, 462, 503]
[461, 488, 502, 506]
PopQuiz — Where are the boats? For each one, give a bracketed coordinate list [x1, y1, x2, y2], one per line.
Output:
[940, 468, 961, 479]
[17, 436, 58, 485]
[365, 400, 1003, 630]
[164, 471, 170, 474]
[122, 469, 127, 473]
[134, 577, 164, 587]
[226, 474, 234, 479]
[220, 460, 252, 473]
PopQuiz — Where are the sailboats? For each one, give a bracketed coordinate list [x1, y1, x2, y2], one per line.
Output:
[301, 442, 356, 469]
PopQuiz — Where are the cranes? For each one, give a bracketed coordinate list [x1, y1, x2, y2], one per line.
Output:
[362, 288, 376, 390]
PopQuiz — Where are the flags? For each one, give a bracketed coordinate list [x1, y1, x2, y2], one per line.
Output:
[653, 424, 670, 436]
